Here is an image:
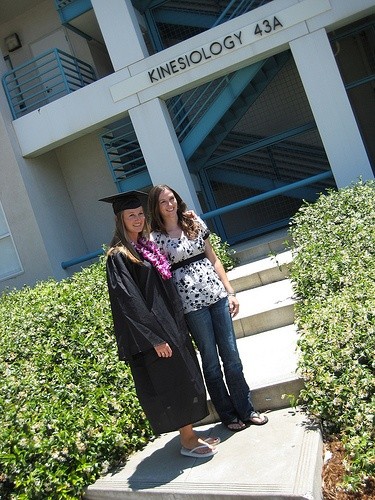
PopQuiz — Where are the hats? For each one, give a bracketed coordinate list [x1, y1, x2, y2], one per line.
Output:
[98, 191, 150, 215]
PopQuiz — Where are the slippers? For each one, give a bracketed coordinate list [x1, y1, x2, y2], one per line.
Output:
[181, 436, 221, 457]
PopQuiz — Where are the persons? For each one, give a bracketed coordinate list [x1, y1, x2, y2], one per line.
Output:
[147, 184, 268, 431]
[106, 190, 221, 457]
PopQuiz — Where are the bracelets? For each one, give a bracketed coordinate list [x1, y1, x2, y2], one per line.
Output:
[228, 293, 236, 297]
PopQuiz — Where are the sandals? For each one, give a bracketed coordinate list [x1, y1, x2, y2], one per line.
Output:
[243, 411, 269, 425]
[224, 418, 248, 431]
